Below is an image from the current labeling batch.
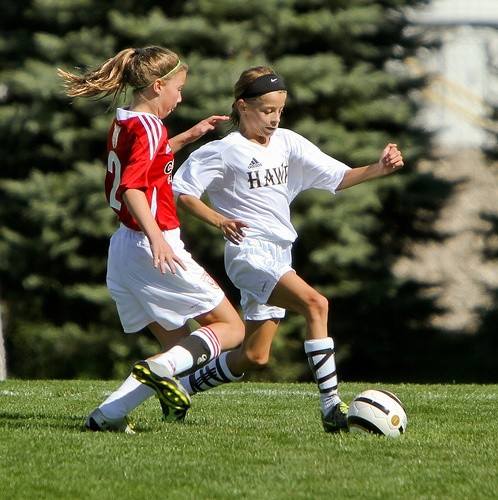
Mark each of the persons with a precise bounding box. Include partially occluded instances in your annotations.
[57,43,245,436]
[153,66,404,435]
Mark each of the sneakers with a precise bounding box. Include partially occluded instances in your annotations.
[85,407,143,436]
[157,396,186,423]
[130,360,192,412]
[319,401,348,433]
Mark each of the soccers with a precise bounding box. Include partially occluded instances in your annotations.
[347,389,407,436]
